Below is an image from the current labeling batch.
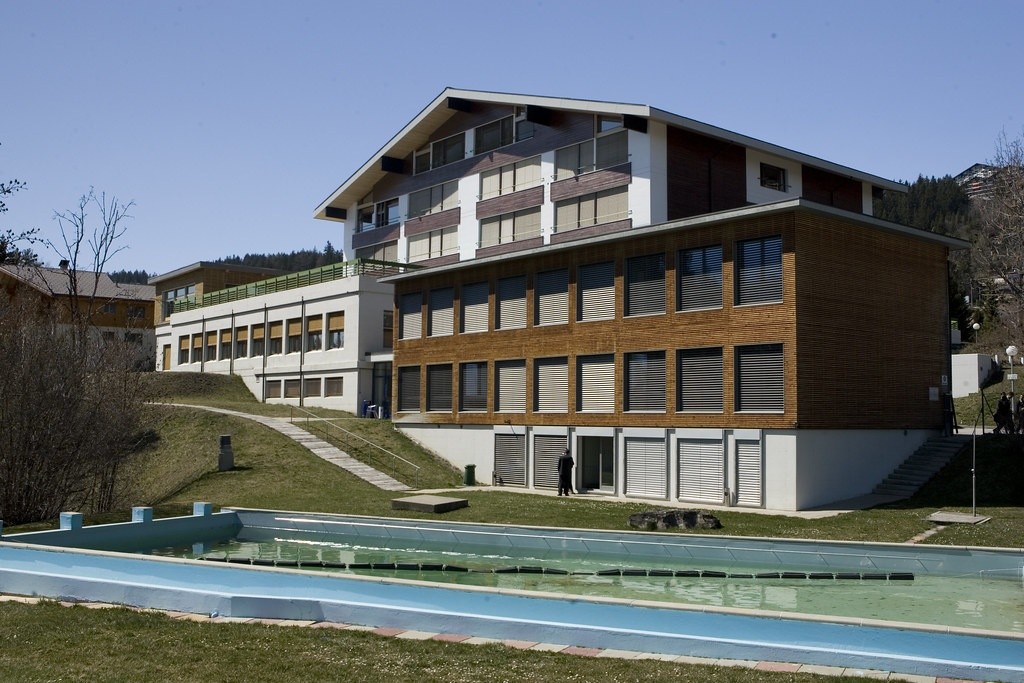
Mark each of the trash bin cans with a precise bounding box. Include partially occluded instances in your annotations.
[463,463,476,484]
[362,399,372,418]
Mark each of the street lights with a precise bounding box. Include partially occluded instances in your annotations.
[973,322,980,343]
[1005,345,1019,423]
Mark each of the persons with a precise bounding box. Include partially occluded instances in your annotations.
[994,392,1024,434]
[557,448,575,496]
